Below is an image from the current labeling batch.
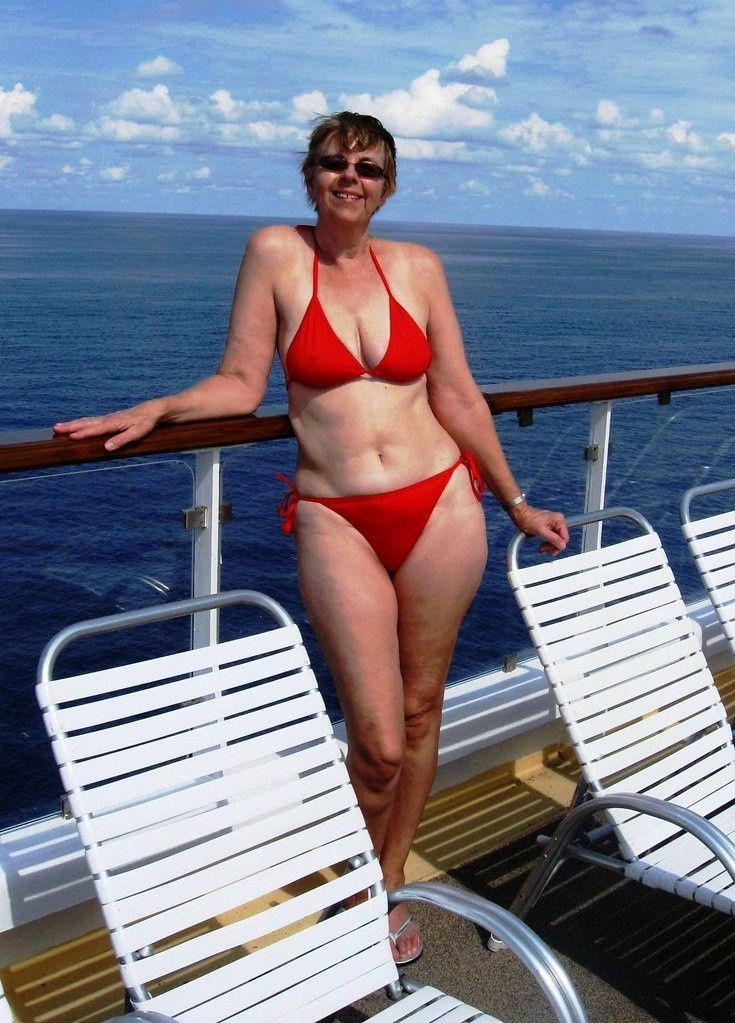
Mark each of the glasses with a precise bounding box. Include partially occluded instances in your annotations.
[310,155,388,178]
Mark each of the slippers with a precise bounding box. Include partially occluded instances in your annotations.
[388,913,423,966]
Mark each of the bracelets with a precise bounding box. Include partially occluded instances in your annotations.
[504,493,526,512]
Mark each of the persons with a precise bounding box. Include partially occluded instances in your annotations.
[51,114,572,966]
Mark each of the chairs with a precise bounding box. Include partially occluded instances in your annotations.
[680,478,735,655]
[485,507,735,953]
[33,593,584,1023]
